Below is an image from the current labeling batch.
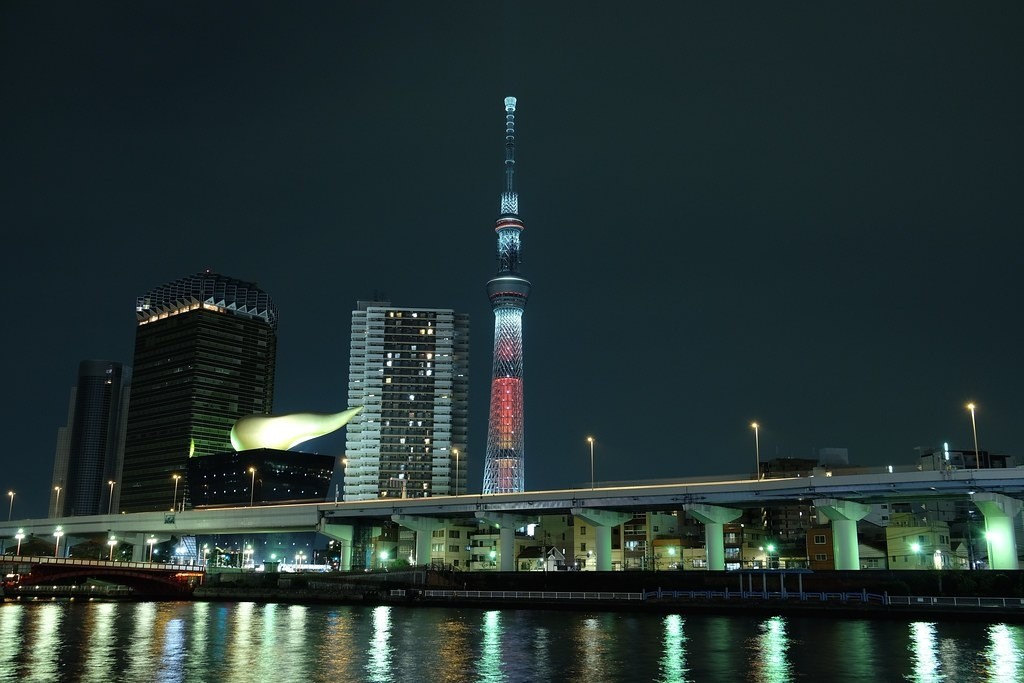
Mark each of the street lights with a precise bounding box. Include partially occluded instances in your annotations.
[295,550,306,567]
[751,422,760,480]
[14,528,25,555]
[768,544,775,568]
[107,535,117,560]
[488,551,497,569]
[452,447,458,496]
[52,525,65,557]
[983,530,998,570]
[108,480,117,515]
[147,534,158,563]
[244,544,254,567]
[8,491,16,520]
[588,437,593,490]
[342,458,348,502]
[172,474,182,511]
[909,542,920,571]
[55,486,62,518]
[248,467,255,506]
[667,546,676,563]
[379,550,388,569]
[202,544,209,565]
[966,403,981,468]
[176,546,188,565]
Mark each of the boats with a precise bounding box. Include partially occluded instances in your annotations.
[368,585,427,609]
